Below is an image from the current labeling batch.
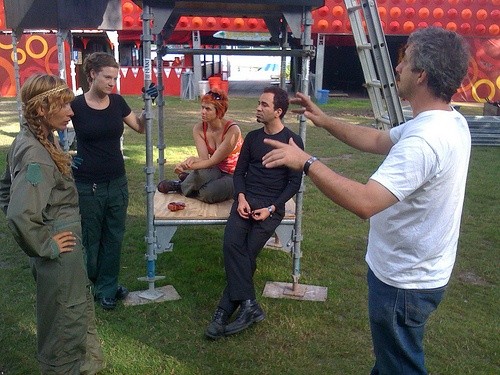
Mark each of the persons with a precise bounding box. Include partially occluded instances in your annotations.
[261,26,471,375]
[204,86,304,339]
[157,88,244,204]
[69,52,158,310]
[0,74,105,375]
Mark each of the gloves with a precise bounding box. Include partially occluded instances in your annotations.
[141,83,158,101]
[70,156,84,169]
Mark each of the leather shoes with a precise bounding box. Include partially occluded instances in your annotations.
[205,307,229,339]
[224,298,264,334]
[178,172,189,181]
[158,179,181,193]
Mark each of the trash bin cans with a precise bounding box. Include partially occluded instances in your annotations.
[317,90,330,104]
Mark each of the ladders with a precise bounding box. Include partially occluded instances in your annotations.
[341,0,409,130]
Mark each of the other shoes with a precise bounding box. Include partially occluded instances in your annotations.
[95,295,116,309]
[113,286,128,300]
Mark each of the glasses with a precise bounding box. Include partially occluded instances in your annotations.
[205,91,221,100]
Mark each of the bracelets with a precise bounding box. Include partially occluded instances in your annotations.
[268,206,273,217]
[304,157,319,176]
